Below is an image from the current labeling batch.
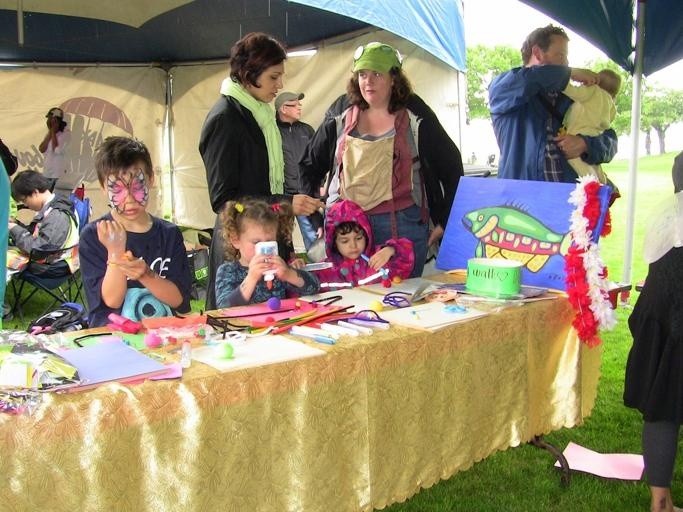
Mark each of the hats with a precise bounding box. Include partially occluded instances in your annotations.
[274,92,304,110]
[352,43,402,76]
[45,107,64,119]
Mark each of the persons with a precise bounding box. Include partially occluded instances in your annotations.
[198,31,327,217]
[78,136,191,328]
[288,199,416,297]
[214,201,321,309]
[2,170,80,321]
[296,41,465,279]
[274,92,327,253]
[38,107,72,178]
[622,150,683,512]
[487,23,618,183]
[562,69,620,184]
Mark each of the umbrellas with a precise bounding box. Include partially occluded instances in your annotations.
[59,96,133,157]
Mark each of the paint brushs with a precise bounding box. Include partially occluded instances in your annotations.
[360,253,388,275]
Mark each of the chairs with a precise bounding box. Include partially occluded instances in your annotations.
[0,193,90,324]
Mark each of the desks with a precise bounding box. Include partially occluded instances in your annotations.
[0,270,604,512]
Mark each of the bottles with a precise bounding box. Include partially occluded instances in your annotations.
[256,241,279,290]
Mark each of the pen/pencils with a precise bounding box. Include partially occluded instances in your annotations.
[250,305,390,344]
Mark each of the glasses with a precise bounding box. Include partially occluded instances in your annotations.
[286,103,302,108]
[21,193,30,204]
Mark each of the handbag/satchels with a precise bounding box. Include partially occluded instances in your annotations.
[0,140,18,176]
[28,302,89,335]
